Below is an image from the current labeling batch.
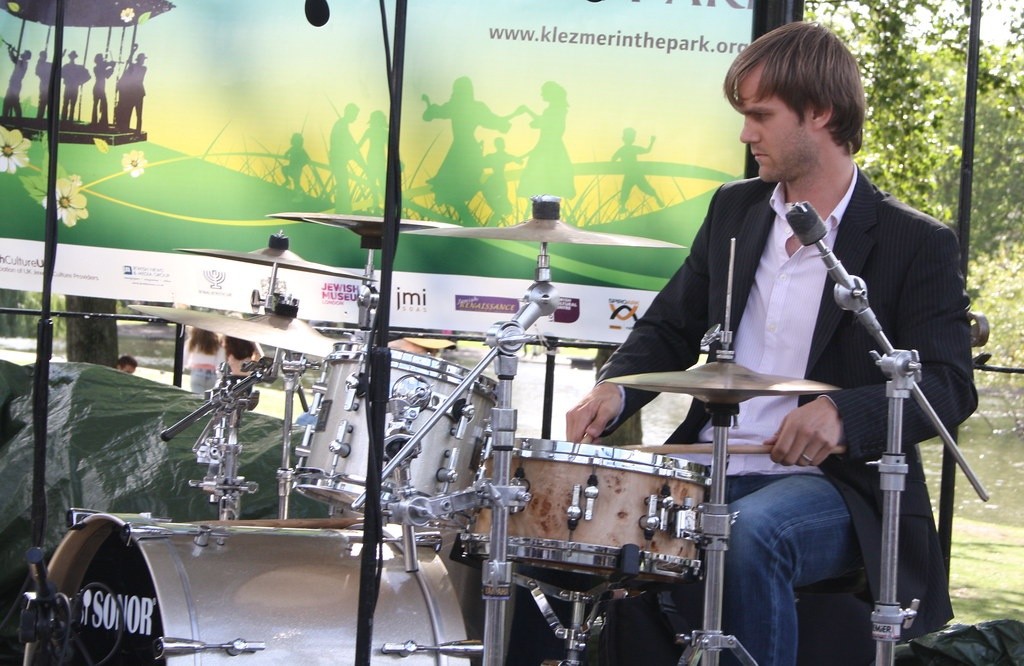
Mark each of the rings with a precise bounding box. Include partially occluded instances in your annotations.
[802,454,811,464]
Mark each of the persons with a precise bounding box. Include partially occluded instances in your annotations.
[565,21,980,666]
[115,356,138,374]
[183,312,265,397]
[389,336,456,357]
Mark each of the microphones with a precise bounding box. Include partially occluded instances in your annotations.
[786,201,853,288]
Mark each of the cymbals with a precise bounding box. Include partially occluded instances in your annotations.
[602,361,842,407]
[127,305,338,358]
[173,246,379,284]
[265,213,464,239]
[399,220,686,251]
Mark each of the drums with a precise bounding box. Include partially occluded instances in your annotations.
[23,513,473,666]
[291,341,499,529]
[461,438,712,581]
